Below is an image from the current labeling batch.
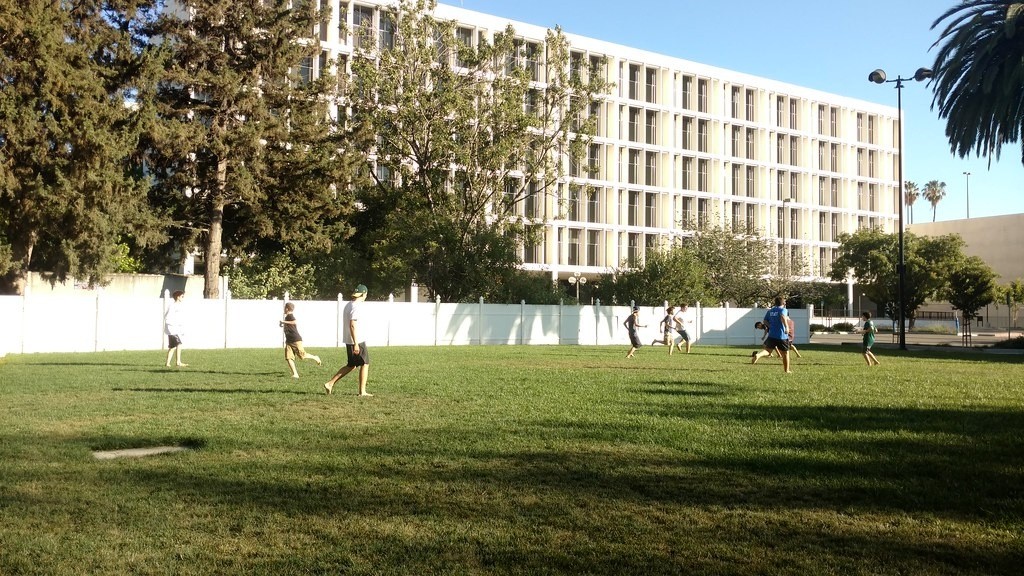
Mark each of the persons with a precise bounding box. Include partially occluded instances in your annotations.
[652,306,675,355]
[787,311,802,358]
[623,306,647,359]
[164,290,189,367]
[856,311,880,366]
[324,285,373,396]
[754,322,782,358]
[673,303,693,353]
[751,297,791,373]
[280,302,322,378]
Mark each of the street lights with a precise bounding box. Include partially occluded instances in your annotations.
[781,196,796,298]
[963,171,972,220]
[858,292,866,327]
[867,67,935,352]
[568,270,587,305]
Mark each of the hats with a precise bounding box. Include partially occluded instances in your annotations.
[351,284,367,298]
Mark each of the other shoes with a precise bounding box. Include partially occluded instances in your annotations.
[652,339,657,346]
[324,382,332,395]
[677,343,681,351]
[628,351,635,357]
[752,354,759,365]
[314,356,321,365]
[874,361,880,365]
